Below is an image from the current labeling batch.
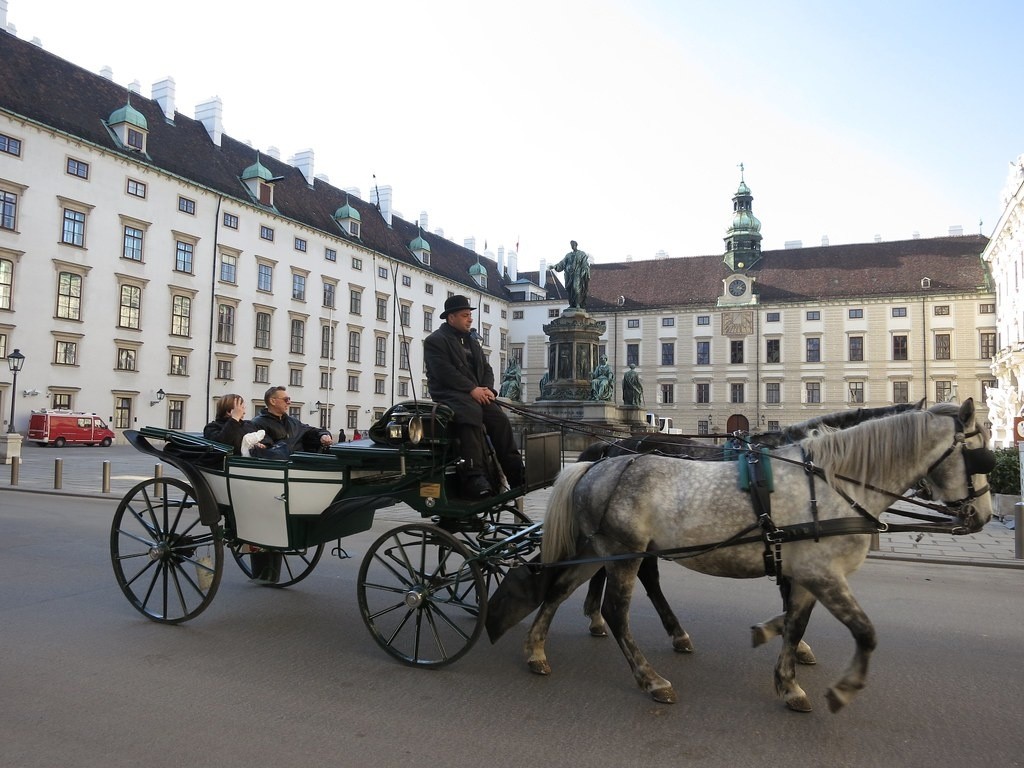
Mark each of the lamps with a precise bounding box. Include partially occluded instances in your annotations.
[708,414,712,425]
[761,415,765,424]
[150,388,165,407]
[310,400,322,415]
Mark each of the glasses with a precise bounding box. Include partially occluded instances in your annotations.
[271,397,291,402]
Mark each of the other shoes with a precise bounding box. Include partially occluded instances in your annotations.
[509,467,527,489]
[464,475,492,499]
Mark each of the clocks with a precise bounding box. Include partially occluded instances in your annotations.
[728,279,747,297]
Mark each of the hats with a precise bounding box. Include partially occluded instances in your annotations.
[440,295,477,319]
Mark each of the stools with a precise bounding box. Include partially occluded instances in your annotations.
[368,399,455,452]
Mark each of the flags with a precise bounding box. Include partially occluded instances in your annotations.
[516,242,519,252]
[485,241,488,249]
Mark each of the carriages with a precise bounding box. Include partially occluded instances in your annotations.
[108,377,996,712]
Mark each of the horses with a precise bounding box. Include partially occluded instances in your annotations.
[526,395,997,712]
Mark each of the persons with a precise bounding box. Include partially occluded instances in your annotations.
[338,429,346,443]
[548,240,590,308]
[498,356,522,400]
[353,430,361,440]
[252,387,332,453]
[424,295,527,497]
[588,354,614,401]
[622,363,643,405]
[203,394,272,457]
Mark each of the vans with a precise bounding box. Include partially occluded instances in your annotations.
[26,409,115,447]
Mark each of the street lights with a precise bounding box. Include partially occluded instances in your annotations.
[0,349,25,465]
